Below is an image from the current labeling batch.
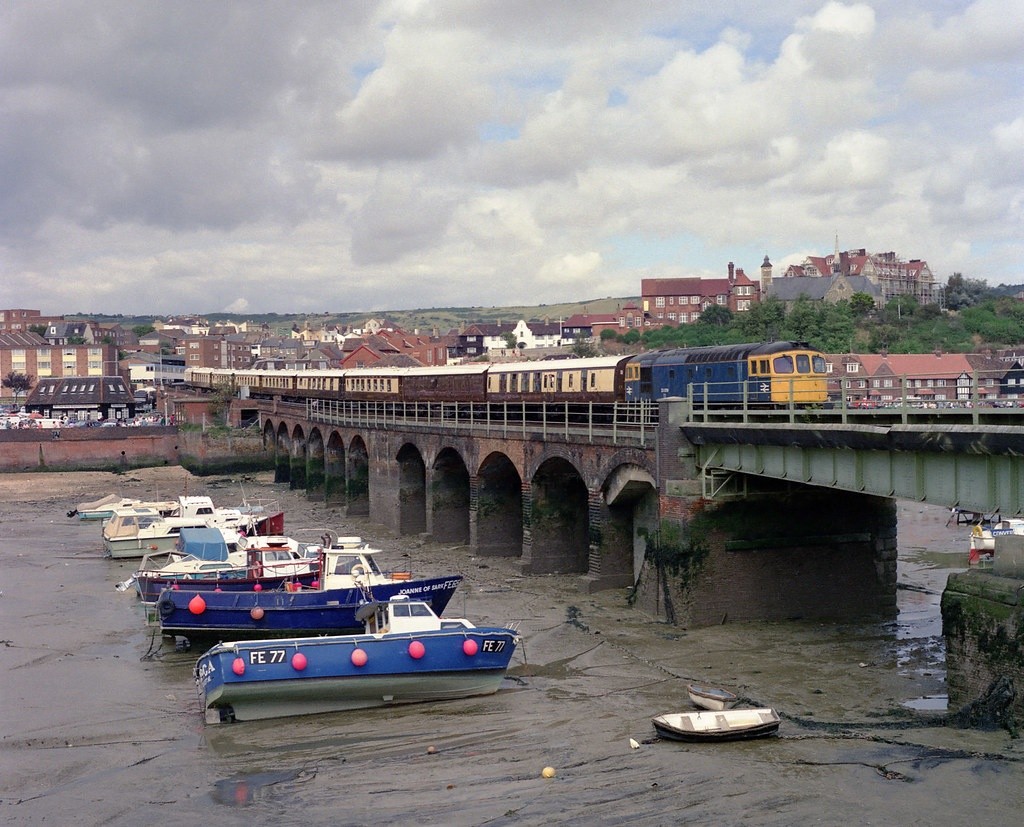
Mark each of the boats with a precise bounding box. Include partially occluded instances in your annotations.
[650,706,783,743]
[687,682,739,711]
[64,476,524,724]
[969,518,1024,562]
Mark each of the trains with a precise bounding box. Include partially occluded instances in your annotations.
[183,338,830,408]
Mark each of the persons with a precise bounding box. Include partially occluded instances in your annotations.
[4,413,176,430]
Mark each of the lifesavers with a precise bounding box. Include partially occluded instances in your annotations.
[389,569,413,582]
